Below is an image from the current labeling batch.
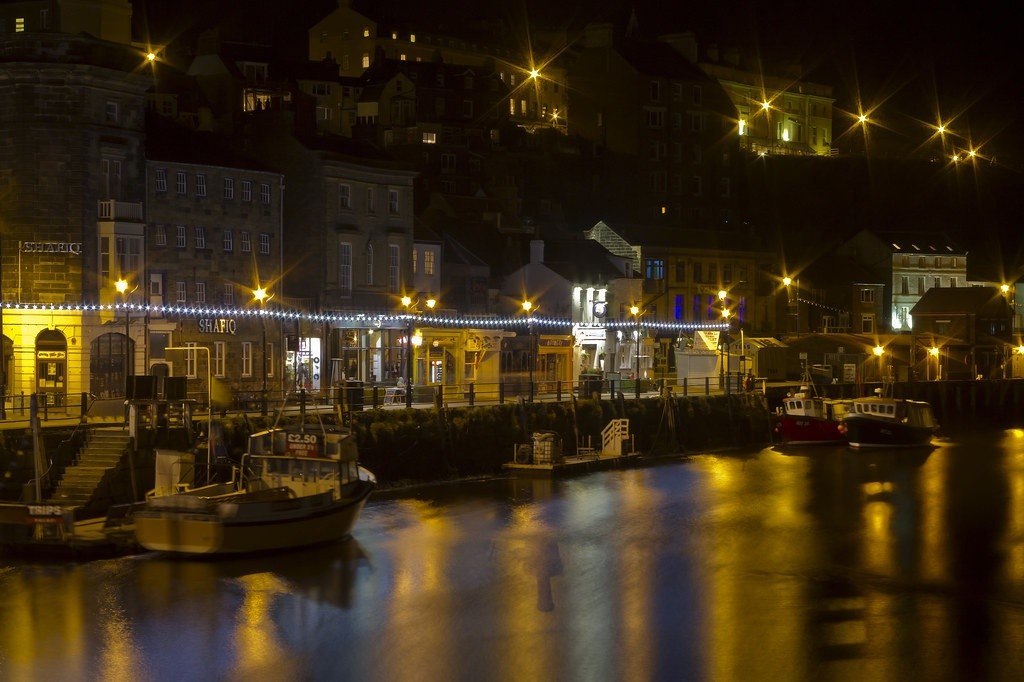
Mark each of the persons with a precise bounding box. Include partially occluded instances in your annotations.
[298,362,306,385]
[396,377,414,396]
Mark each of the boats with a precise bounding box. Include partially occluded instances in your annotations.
[834,366,943,453]
[130,337,378,555]
[772,351,842,448]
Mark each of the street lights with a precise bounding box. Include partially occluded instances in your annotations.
[401,293,414,407]
[630,300,645,399]
[716,288,730,393]
[113,277,131,425]
[519,300,537,402]
[253,284,270,417]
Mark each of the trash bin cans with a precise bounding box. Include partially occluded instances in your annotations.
[342,381,364,412]
[578,374,603,400]
